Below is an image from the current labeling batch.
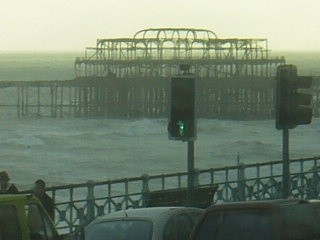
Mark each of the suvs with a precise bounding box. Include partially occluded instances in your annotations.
[0,194,60,240]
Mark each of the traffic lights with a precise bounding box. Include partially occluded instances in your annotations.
[168,78,196,139]
[276,65,310,130]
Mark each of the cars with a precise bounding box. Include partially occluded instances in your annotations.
[84,207,205,240]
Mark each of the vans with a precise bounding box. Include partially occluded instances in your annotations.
[189,200,320,240]
